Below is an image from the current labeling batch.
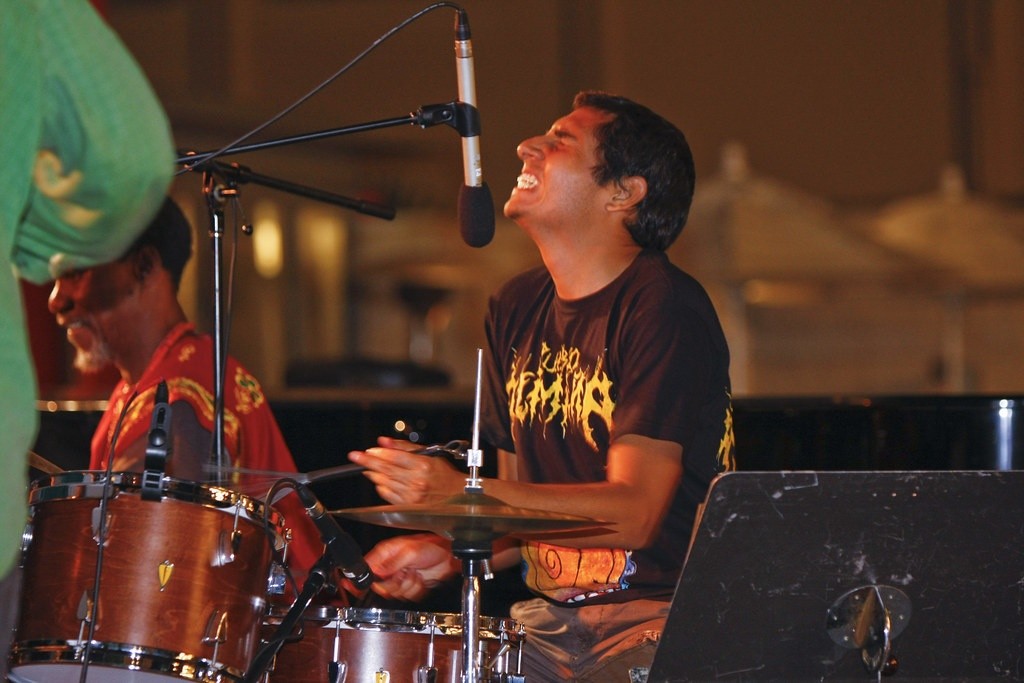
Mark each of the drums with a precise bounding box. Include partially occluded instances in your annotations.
[254,598,527,683]
[8,467,293,683]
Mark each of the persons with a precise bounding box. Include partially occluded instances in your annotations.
[46,192,322,606]
[348,91,738,683]
[0,0,176,683]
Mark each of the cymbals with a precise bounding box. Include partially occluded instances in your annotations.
[325,488,619,540]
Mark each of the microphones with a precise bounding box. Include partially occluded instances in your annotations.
[294,482,376,591]
[454,8,495,248]
[144,380,171,467]
